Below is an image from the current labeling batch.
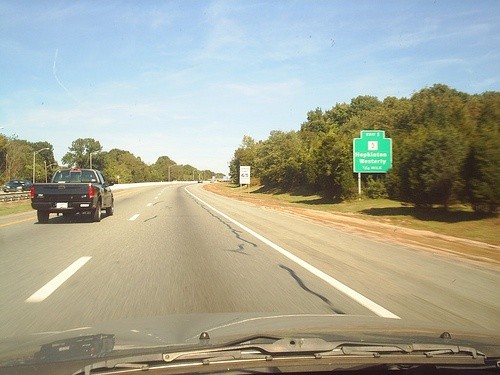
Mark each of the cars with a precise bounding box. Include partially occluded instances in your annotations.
[1,312,500,374]
[2,179,32,193]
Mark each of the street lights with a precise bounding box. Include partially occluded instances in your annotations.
[90,152,97,169]
[33,147,49,183]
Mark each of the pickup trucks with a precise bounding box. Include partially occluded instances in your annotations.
[31,168,114,222]
